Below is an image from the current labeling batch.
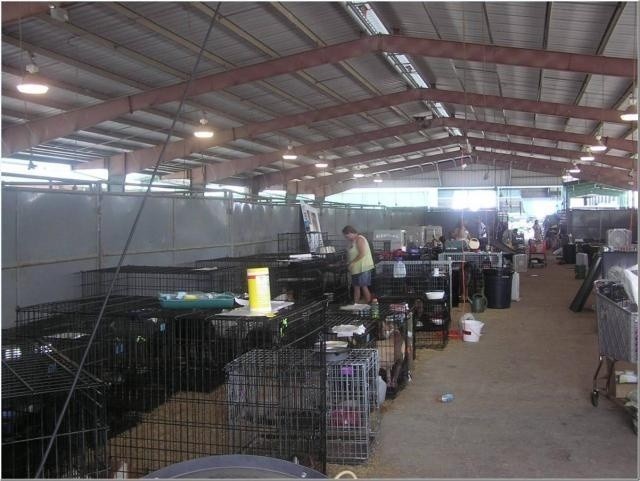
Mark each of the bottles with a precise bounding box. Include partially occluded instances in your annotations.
[371,298,380,319]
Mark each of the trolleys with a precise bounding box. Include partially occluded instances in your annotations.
[589,276,639,427]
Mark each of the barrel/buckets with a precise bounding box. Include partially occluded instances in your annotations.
[462,319,484,342]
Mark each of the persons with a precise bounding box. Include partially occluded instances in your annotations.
[449,221,519,243]
[531,219,542,241]
[341,225,375,304]
